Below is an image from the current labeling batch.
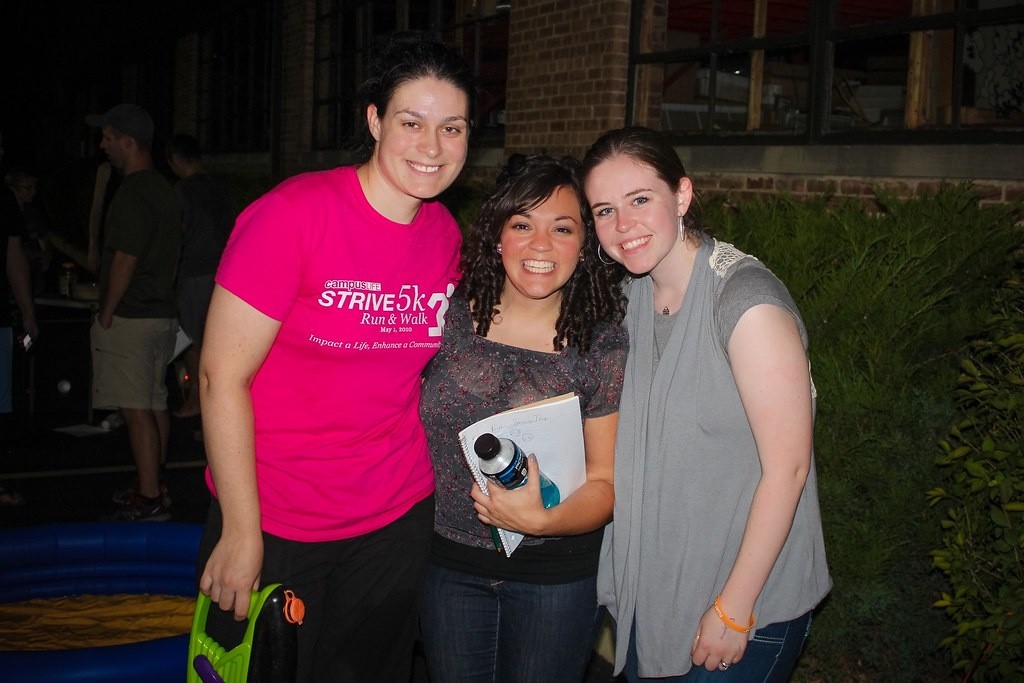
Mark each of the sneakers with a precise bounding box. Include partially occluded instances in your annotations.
[113,483,180,524]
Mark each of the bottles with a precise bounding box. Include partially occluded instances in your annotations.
[57,262,78,297]
[474,434,560,512]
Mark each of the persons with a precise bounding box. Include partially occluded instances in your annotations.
[581,126,833,683]
[0,104,224,524]
[198,40,480,683]
[417,153,631,683]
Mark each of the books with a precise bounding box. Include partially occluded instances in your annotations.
[458,391,587,558]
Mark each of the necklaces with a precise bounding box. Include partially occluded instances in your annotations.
[652,295,684,316]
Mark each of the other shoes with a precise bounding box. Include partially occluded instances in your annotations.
[1,486,28,509]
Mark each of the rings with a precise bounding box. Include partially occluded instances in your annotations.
[721,659,729,668]
[695,635,699,639]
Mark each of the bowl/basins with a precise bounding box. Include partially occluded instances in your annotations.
[69,284,100,300]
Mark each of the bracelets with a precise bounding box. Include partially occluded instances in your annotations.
[714,596,754,633]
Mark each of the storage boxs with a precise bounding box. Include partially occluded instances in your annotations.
[658,60,905,112]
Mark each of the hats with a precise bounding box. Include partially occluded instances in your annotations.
[85,103,154,143]
